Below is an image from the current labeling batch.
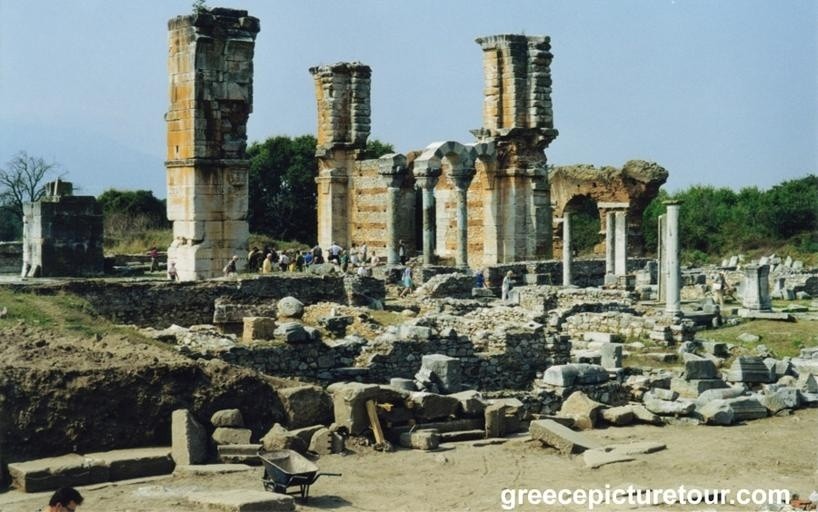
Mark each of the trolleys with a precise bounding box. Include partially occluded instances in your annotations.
[256,449,342,503]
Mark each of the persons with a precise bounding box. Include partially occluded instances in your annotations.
[475,269,488,290]
[398,238,407,265]
[399,262,416,298]
[501,270,514,301]
[41,485,84,511]
[149,245,161,272]
[169,260,179,281]
[223,242,378,274]
